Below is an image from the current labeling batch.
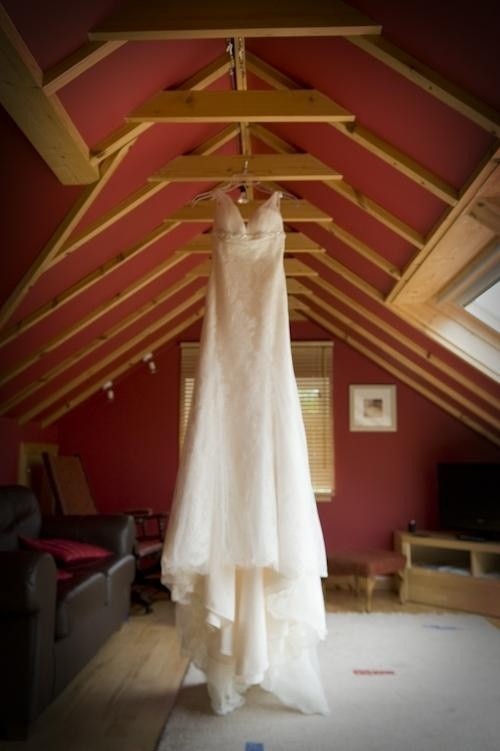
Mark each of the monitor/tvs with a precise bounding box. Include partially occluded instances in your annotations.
[432,456,500,540]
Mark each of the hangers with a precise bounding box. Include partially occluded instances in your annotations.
[187,159,301,212]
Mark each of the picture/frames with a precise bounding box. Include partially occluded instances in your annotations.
[348,382,398,434]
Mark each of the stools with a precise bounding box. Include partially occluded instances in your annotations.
[323,546,408,612]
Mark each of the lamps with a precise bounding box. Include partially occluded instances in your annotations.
[141,351,158,374]
[102,381,115,400]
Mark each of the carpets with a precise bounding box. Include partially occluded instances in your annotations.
[153,607,499,751]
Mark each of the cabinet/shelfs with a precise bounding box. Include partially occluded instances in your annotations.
[391,526,500,621]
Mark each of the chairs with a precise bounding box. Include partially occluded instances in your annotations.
[0,484,140,751]
[40,449,173,619]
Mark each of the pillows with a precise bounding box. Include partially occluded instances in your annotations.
[14,527,114,572]
[52,569,84,585]
